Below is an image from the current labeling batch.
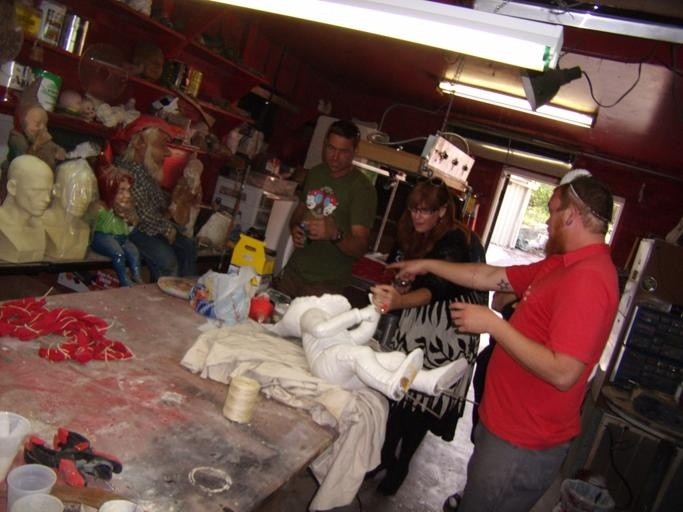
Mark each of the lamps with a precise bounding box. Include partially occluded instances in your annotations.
[437,79,593,127]
[209,1,563,72]
[522,56,648,110]
[447,127,575,170]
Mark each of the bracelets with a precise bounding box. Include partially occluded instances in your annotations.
[330,229,345,246]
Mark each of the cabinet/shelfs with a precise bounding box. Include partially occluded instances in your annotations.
[213,176,298,276]
[0,0,271,165]
[350,133,476,294]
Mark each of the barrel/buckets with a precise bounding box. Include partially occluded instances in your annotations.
[197,210,232,251]
[37,67,61,114]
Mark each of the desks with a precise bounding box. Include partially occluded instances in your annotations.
[1,285,338,512]
[0,250,221,276]
[577,384,683,512]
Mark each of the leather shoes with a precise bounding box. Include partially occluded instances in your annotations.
[365,454,408,496]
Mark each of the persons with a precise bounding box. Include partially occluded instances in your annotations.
[288,288,467,403]
[361,174,486,497]
[279,119,378,301]
[383,173,620,511]
[0,102,204,285]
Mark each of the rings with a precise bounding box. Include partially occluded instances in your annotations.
[380,303,388,312]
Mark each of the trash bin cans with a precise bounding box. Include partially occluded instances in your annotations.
[560,479,614,512]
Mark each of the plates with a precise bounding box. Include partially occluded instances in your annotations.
[157,276,193,299]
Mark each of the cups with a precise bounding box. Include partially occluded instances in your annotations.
[8,495,65,512]
[98,498,138,511]
[5,463,56,509]
[0,410,30,482]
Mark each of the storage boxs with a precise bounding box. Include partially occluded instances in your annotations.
[228,234,277,289]
[252,171,298,196]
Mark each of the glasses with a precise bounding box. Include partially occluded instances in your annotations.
[416,174,444,188]
[408,204,436,214]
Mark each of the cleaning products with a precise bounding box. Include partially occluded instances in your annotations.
[143,45,203,97]
